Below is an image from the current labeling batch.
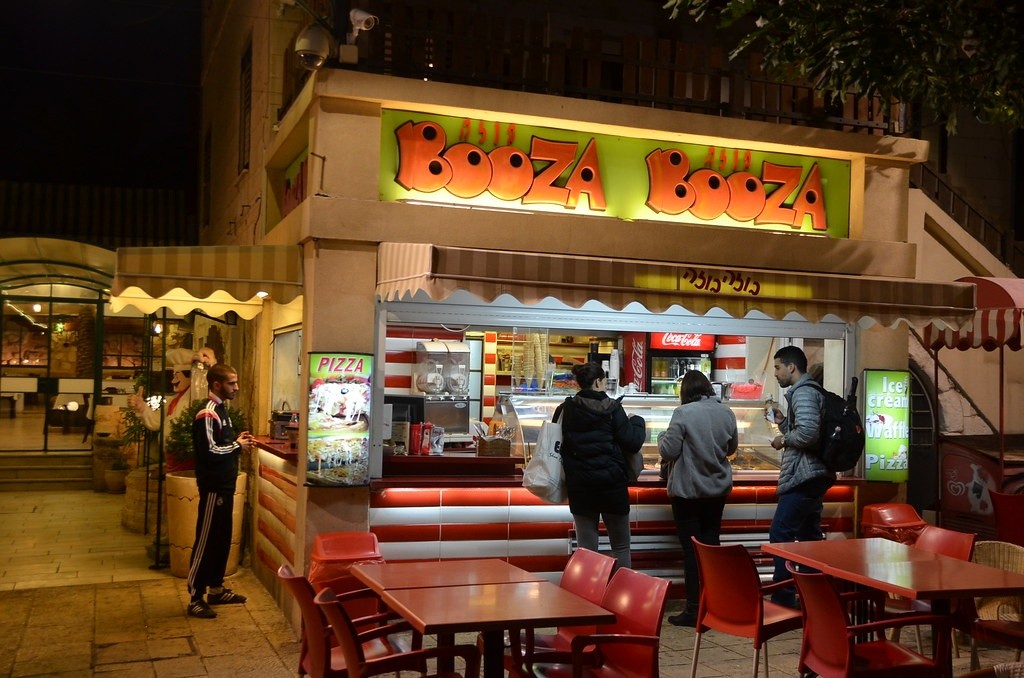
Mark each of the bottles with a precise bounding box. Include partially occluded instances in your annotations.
[652,354,712,400]
[288,410,298,426]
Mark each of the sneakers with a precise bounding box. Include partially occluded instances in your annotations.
[207,589,247,607]
[187,599,217,618]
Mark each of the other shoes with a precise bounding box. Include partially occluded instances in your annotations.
[668,612,697,628]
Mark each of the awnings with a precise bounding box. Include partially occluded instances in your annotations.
[376,240,978,333]
[923,274,1024,495]
[109,244,304,322]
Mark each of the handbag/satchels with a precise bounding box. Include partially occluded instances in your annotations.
[659,457,672,478]
[522,408,566,504]
[618,449,644,485]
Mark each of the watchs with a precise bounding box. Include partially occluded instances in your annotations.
[780,437,786,446]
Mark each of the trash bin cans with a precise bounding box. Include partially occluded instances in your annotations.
[860,503,927,546]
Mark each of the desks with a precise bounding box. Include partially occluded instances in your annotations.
[379,580,616,678]
[58,379,144,435]
[822,559,1021,678]
[350,557,548,675]
[760,538,933,677]
[1,377,38,417]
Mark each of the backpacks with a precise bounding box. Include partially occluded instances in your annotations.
[790,383,864,472]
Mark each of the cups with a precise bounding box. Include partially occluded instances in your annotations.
[602,348,619,380]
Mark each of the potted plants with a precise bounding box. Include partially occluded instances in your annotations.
[105,455,131,491]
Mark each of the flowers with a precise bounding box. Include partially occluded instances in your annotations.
[169,404,253,478]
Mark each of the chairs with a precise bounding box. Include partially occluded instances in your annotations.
[690,525,1024,678]
[278,547,673,678]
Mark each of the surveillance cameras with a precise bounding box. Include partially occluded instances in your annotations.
[350,9,380,29]
[295,21,331,71]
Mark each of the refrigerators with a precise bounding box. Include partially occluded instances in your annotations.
[621,331,719,467]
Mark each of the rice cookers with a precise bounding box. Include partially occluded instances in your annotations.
[268,401,299,440]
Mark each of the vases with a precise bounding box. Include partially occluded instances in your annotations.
[166,469,249,578]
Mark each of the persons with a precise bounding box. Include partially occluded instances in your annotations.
[657,370,740,626]
[127,348,218,545]
[762,345,840,608]
[552,362,647,573]
[187,363,261,617]
